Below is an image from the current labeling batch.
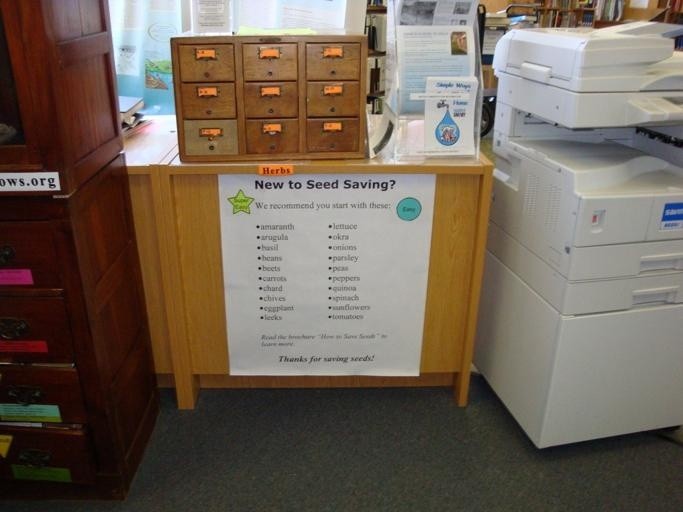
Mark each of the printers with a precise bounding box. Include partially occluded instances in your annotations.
[473,19,683,451]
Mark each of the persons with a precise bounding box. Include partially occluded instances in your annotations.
[451,33,467,54]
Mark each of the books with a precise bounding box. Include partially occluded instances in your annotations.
[546,1,593,28]
[484,4,538,28]
